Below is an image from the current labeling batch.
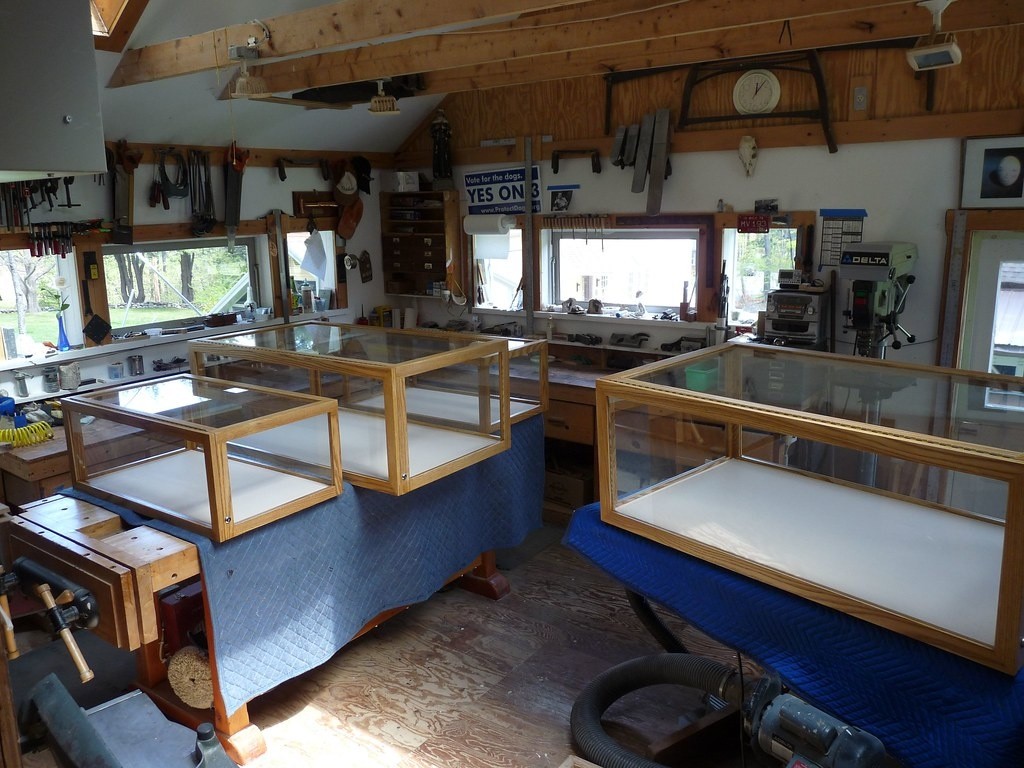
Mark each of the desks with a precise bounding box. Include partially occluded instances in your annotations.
[0,332,510,518]
[342,333,787,507]
[562,500,1024,768]
[0,375,549,766]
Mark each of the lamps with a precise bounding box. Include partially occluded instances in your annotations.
[368,77,400,116]
[229,44,273,98]
[907,0,961,72]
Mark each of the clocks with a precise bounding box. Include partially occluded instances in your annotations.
[733,69,780,116]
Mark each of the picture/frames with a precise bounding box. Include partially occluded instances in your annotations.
[958,133,1024,210]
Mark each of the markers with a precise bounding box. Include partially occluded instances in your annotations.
[666,312,679,321]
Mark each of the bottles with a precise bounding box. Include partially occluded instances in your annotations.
[289,276,301,309]
[244,286,256,321]
[301,278,313,312]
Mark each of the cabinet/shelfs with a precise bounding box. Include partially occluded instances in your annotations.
[188,320,512,496]
[337,324,551,433]
[592,340,1024,675]
[378,191,463,299]
[59,372,344,544]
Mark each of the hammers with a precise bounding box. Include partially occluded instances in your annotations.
[299,198,339,213]
[257,208,294,324]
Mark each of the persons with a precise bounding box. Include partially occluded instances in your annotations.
[553,191,568,212]
